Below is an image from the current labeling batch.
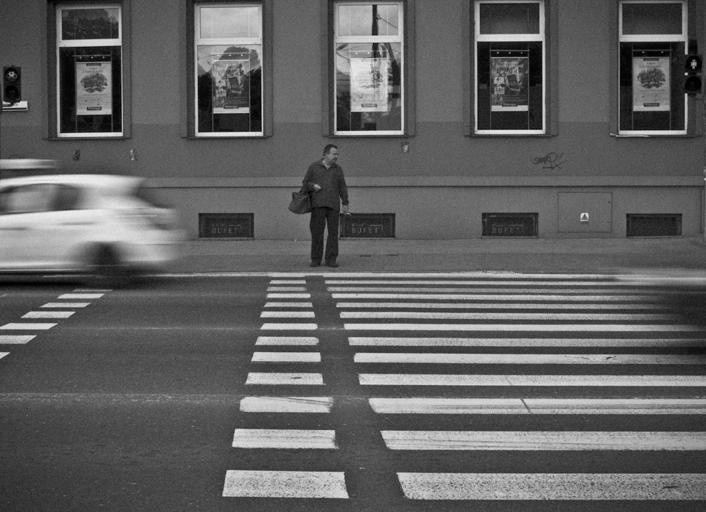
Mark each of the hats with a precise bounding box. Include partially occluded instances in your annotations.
[1,175,188,286]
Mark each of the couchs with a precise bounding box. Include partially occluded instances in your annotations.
[683,53,702,95]
[4,64,23,103]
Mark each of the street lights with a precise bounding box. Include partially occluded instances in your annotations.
[289,191,312,213]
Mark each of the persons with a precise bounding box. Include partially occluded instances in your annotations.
[302,145,349,268]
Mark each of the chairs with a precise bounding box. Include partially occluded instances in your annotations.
[310,262,320,266]
[325,263,339,267]
